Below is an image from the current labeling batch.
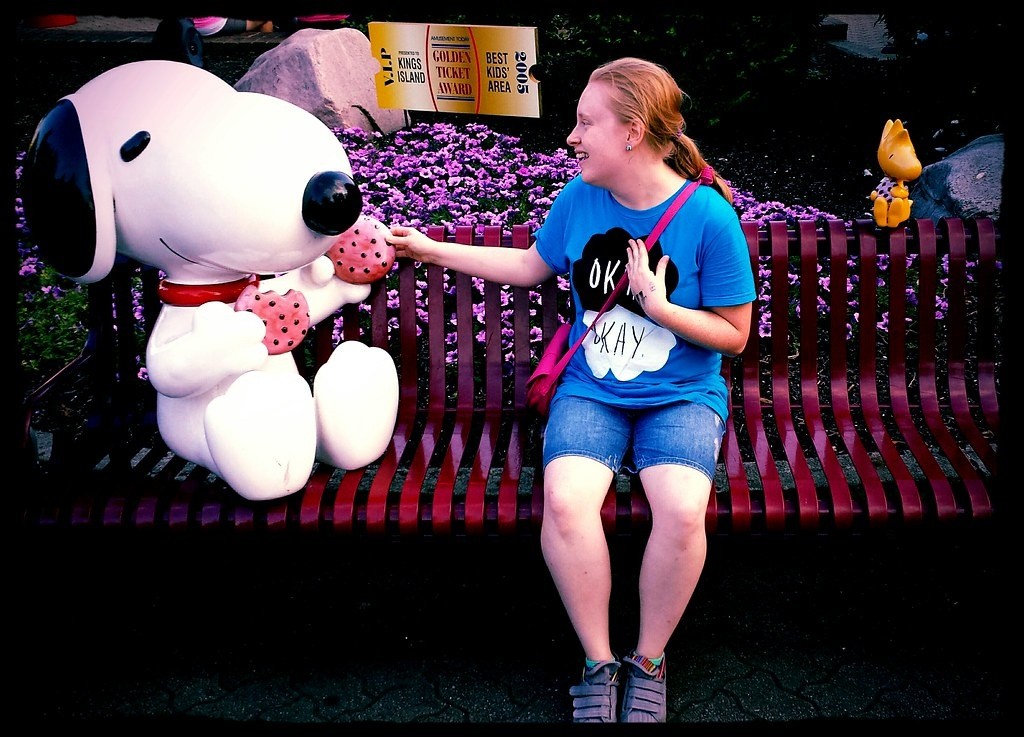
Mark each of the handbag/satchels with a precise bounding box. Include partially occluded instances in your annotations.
[525,323,571,418]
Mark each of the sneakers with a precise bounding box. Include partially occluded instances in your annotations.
[622,649,667,722]
[569,650,622,723]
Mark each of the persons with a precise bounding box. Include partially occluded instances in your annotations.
[193,16,273,36]
[385,58,757,723]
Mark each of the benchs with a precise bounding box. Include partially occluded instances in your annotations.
[17,217,1003,556]
